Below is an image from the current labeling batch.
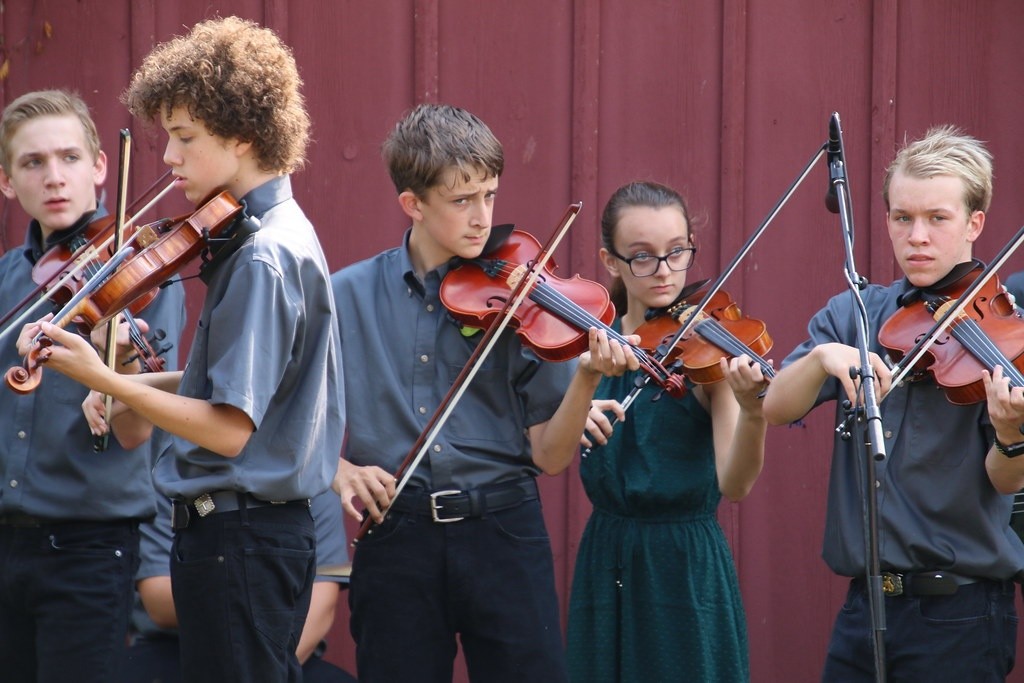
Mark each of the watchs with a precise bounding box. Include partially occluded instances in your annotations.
[993,432,1024,459]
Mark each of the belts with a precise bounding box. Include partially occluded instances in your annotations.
[377,476,540,523]
[1,513,60,530]
[169,490,312,529]
[866,567,982,598]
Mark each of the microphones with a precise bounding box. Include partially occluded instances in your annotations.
[826,112,849,213]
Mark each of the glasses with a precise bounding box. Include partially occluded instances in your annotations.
[608,239,697,277]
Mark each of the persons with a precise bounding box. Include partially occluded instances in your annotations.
[332,98,638,683]
[141,423,359,682]
[764,124,1023,683]
[14,20,333,683]
[1,91,187,683]
[566,184,770,682]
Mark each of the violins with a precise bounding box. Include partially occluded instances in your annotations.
[3,188,245,397]
[628,288,809,432]
[29,211,176,374]
[878,254,1022,408]
[438,225,687,406]
